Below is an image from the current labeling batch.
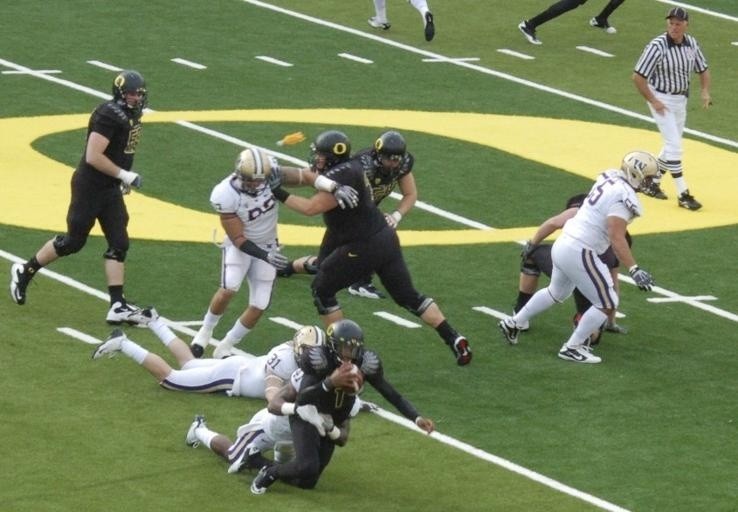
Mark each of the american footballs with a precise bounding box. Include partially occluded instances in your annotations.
[345,365,363,396]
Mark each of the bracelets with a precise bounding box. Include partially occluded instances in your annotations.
[273,187,290,202]
[391,211,402,222]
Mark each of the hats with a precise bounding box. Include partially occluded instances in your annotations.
[665,7,688,20]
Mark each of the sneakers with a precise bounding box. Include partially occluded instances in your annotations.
[678,189,701,211]
[347,281,387,299]
[643,183,666,199]
[451,309,614,366]
[212,343,234,358]
[276,264,293,279]
[365,11,616,45]
[10,262,158,360]
[189,326,214,358]
[185,415,276,495]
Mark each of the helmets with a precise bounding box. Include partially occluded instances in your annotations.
[309,131,350,172]
[376,131,406,177]
[293,326,326,354]
[620,152,658,193]
[236,148,272,196]
[113,71,147,119]
[325,318,365,369]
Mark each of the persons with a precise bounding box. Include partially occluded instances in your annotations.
[514,194,632,334]
[276,130,417,299]
[272,131,472,365]
[518,0,625,45]
[185,319,434,494]
[497,152,661,363]
[367,0,435,41]
[633,7,712,210]
[189,148,360,359]
[9,70,144,325]
[93,308,327,402]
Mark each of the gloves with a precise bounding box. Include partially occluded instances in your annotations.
[267,245,289,271]
[627,264,655,291]
[384,211,402,229]
[118,167,143,194]
[520,240,536,262]
[295,405,318,425]
[602,323,626,334]
[332,183,360,210]
